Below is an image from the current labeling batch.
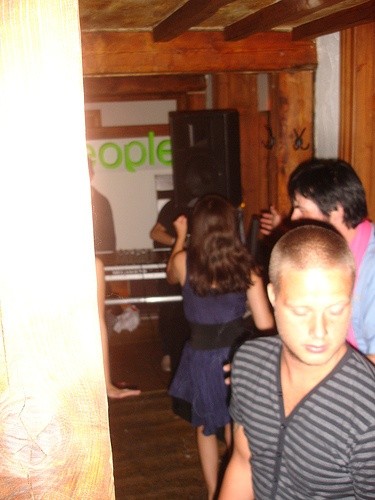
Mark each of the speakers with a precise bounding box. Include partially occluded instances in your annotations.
[169,108,241,212]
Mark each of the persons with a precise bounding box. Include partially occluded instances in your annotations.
[166,195,275,500]
[255,203,281,236]
[217,224,375,499]
[221,217,348,387]
[149,193,203,372]
[94,257,141,399]
[88,155,117,254]
[287,157,375,365]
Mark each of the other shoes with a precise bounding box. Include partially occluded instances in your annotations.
[161,354,172,372]
